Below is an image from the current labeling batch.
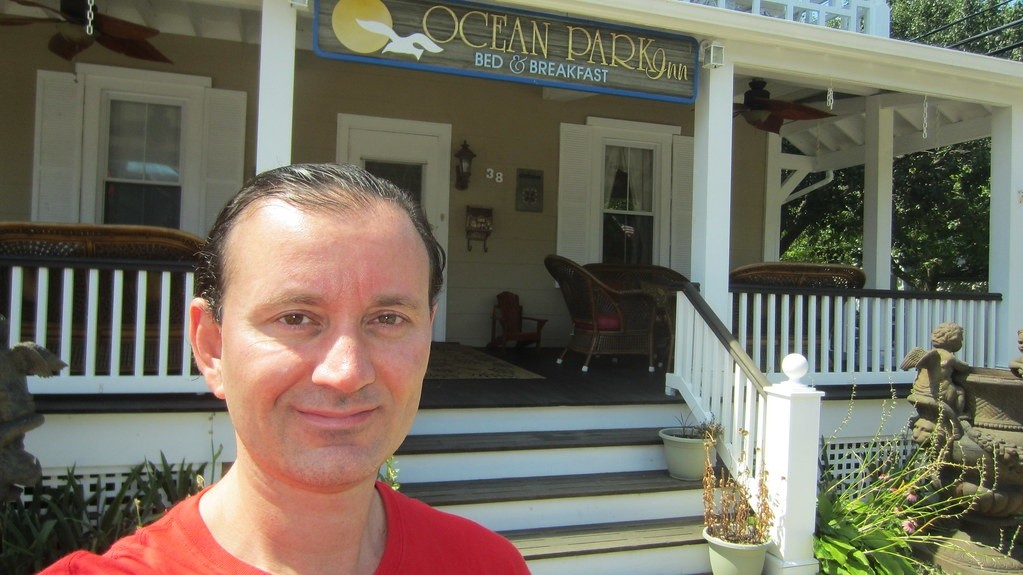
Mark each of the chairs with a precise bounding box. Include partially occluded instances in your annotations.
[0,217,207,364]
[490,291,547,358]
[544,254,661,373]
[584,262,699,349]
[638,278,681,373]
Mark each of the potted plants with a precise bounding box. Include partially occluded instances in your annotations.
[659,404,720,480]
[702,461,771,575]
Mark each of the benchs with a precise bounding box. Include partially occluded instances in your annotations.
[727,260,867,361]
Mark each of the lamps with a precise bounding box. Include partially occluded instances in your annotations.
[455,140,477,191]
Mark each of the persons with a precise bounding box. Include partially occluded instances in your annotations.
[37,161,534,575]
[0,314,68,454]
[899,323,972,429]
[1008,329,1023,379]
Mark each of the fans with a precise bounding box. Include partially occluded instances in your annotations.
[733,75,839,133]
[1,0,176,66]
